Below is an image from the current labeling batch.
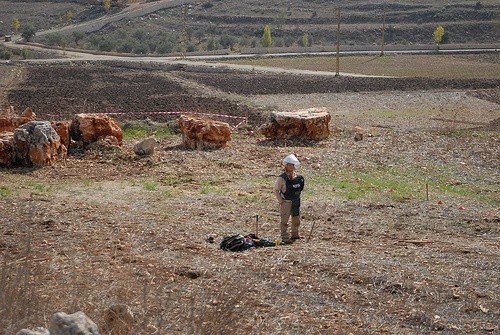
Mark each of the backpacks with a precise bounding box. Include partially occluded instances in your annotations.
[220,234,246,252]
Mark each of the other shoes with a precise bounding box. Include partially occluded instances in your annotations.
[282,239,292,244]
[291,235,305,240]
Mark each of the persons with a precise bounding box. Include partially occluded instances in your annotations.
[274,155,303,243]
